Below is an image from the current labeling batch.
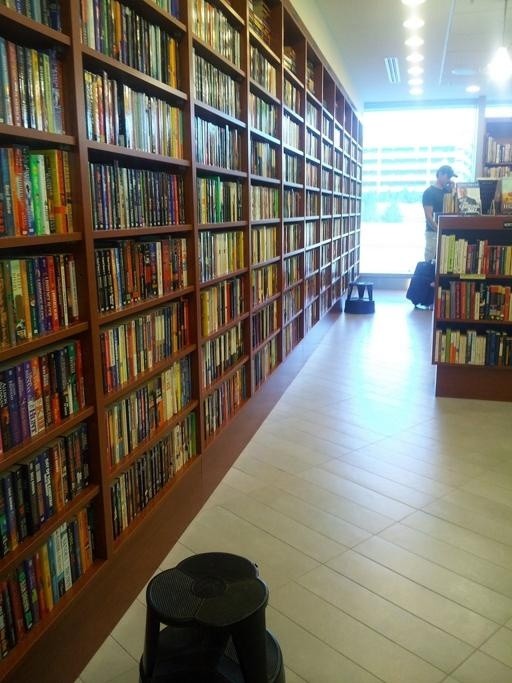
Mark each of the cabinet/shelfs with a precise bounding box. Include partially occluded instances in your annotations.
[472,95,512,214]
[75,1,205,621]
[431,215,512,402]
[0,1,113,683]
[248,1,362,442]
[190,2,250,526]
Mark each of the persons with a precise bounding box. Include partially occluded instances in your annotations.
[457,187,477,215]
[423,165,458,263]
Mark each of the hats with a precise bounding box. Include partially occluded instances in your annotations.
[436,165,458,178]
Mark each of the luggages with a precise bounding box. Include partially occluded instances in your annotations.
[406,251,436,311]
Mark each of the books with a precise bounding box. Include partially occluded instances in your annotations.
[0,145,74,236]
[434,328,512,365]
[489,178,512,215]
[89,163,186,231]
[201,277,247,336]
[82,1,178,88]
[437,280,512,322]
[0,423,89,557]
[3,1,68,34]
[202,322,247,388]
[0,40,66,138]
[193,47,241,118]
[111,411,198,541]
[192,0,241,70]
[84,68,184,160]
[195,116,243,172]
[0,510,94,660]
[197,175,243,223]
[101,298,192,394]
[199,231,246,282]
[439,233,510,276]
[204,366,249,442]
[0,338,86,453]
[95,237,187,314]
[110,355,195,465]
[442,193,457,214]
[483,136,512,178]
[155,0,179,18]
[0,253,79,346]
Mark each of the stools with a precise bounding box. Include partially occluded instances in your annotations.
[345,282,375,313]
[139,553,284,682]
